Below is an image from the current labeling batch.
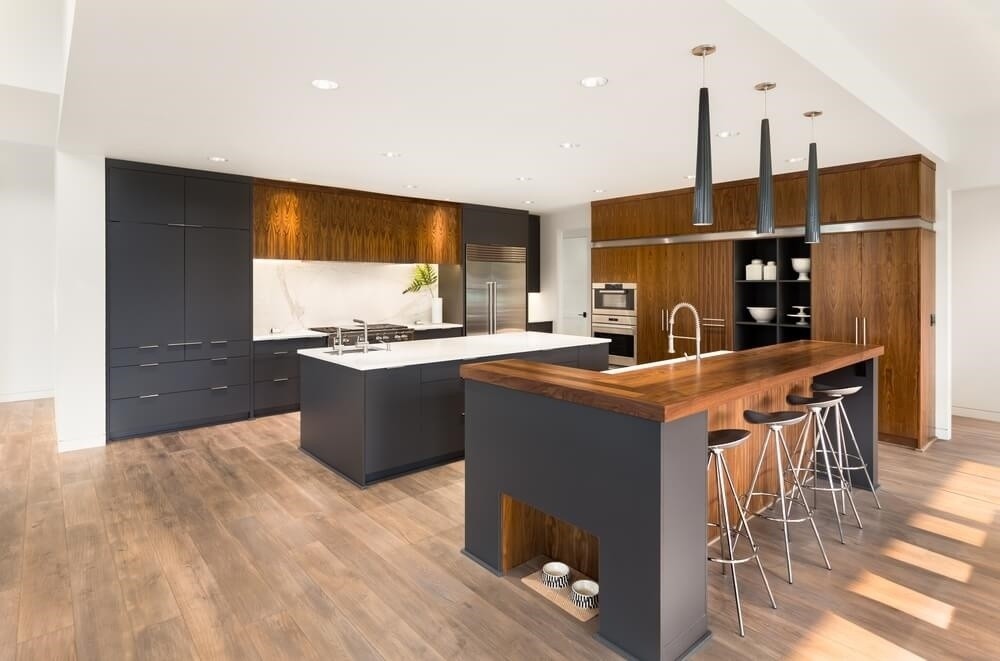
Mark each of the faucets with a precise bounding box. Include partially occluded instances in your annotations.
[667,302,701,361]
[333,328,344,356]
[352,318,369,353]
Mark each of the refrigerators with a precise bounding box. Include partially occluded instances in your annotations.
[466,243,527,338]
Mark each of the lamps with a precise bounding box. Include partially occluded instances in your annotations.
[754,81,777,234]
[806,112,820,244]
[692,45,716,226]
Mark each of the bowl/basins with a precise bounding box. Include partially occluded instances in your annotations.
[746,307,776,322]
[541,561,571,589]
[569,579,599,609]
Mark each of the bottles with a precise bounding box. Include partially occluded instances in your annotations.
[764,262,776,280]
[745,260,764,280]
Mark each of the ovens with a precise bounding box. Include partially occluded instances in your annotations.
[591,281,637,367]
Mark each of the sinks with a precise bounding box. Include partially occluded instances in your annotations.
[600,349,736,375]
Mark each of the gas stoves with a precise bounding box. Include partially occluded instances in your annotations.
[309,322,409,334]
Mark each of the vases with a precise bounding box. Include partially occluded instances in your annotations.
[432,298,442,324]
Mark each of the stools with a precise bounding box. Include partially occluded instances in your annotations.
[705,381,881,637]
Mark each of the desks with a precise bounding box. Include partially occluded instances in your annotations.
[459,339,885,661]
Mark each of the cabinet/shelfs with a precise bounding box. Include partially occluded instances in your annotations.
[636,240,734,365]
[589,153,938,241]
[811,228,936,452]
[104,156,253,442]
[733,235,810,350]
[253,328,611,491]
